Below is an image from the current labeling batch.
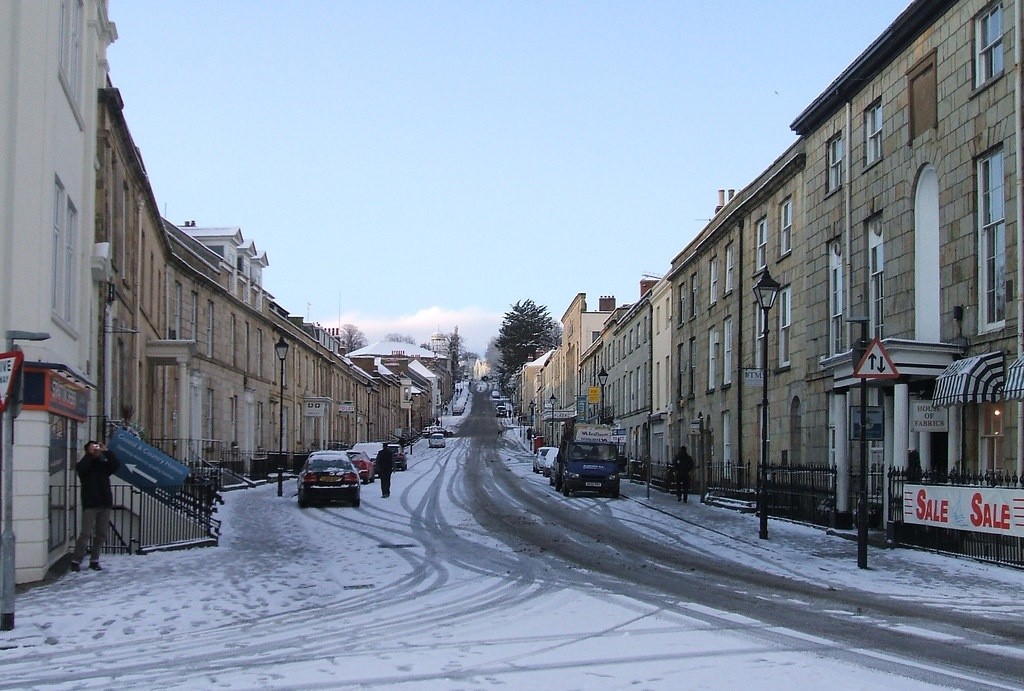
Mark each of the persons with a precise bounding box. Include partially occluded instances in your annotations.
[399,434,404,451]
[375,443,396,498]
[70,441,120,571]
[499,408,532,440]
[672,446,693,503]
[432,418,440,426]
[456,388,463,395]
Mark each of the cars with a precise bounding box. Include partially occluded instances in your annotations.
[496,406,508,418]
[532,446,552,474]
[543,448,559,476]
[352,442,383,475]
[492,391,500,399]
[422,425,453,439]
[549,457,559,486]
[296,449,374,508]
[386,444,408,472]
[428,433,446,448]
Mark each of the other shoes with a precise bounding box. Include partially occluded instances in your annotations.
[678,499,681,502]
[684,500,687,503]
[380,494,390,498]
[89,561,102,572]
[71,562,80,572]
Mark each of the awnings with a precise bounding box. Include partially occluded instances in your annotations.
[931,351,1005,409]
[1002,357,1024,402]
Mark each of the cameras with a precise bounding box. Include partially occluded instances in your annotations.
[93,444,99,449]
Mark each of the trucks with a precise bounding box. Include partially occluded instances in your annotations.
[553,438,629,498]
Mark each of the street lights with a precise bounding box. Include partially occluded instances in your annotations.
[274,334,290,496]
[597,365,609,424]
[427,399,432,435]
[529,401,535,450]
[409,397,414,455]
[365,380,373,444]
[549,393,558,448]
[752,264,783,540]
